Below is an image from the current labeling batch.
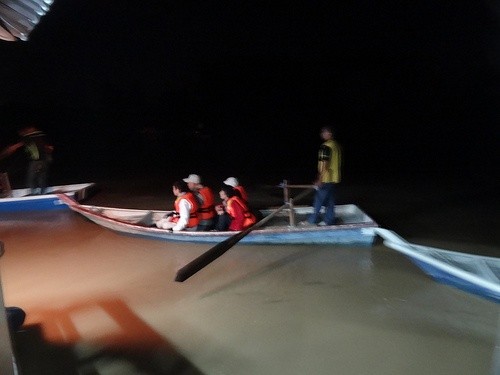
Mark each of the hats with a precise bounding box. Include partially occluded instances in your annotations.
[183,174,201,183]
[223,177,239,187]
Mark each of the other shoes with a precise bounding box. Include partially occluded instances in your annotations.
[300,220,311,225]
[318,221,327,226]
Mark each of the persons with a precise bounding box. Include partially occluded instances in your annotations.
[4,128,53,196]
[183,174,215,230]
[149,180,199,231]
[299,127,341,225]
[208,177,257,231]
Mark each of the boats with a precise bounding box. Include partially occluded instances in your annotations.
[0,182,99,214]
[370,227,500,304]
[54,189,379,245]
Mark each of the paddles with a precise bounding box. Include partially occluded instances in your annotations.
[174,187,315,284]
